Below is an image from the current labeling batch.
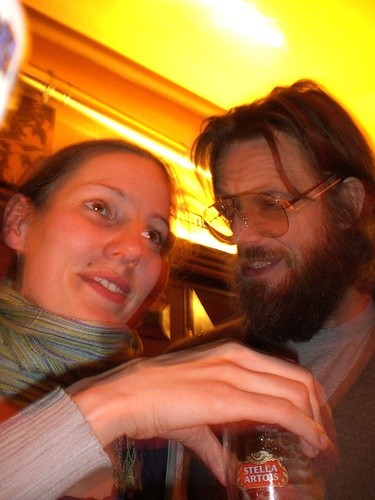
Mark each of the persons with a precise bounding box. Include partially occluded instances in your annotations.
[147,79,375,500]
[0,137,334,500]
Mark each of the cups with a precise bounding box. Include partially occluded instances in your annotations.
[222,420,326,500]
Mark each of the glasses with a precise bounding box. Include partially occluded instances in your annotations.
[201,174,341,244]
